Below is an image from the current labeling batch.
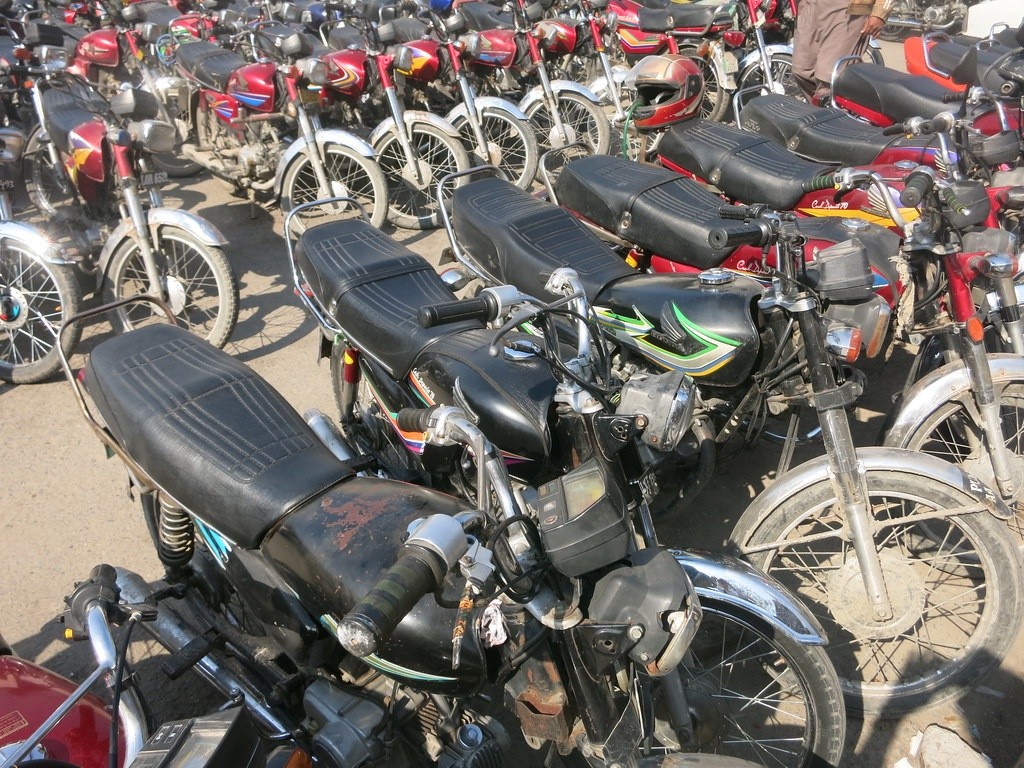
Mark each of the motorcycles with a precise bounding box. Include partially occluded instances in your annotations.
[1,0,1024,768]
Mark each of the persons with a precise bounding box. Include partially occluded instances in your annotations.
[792,0,894,103]
